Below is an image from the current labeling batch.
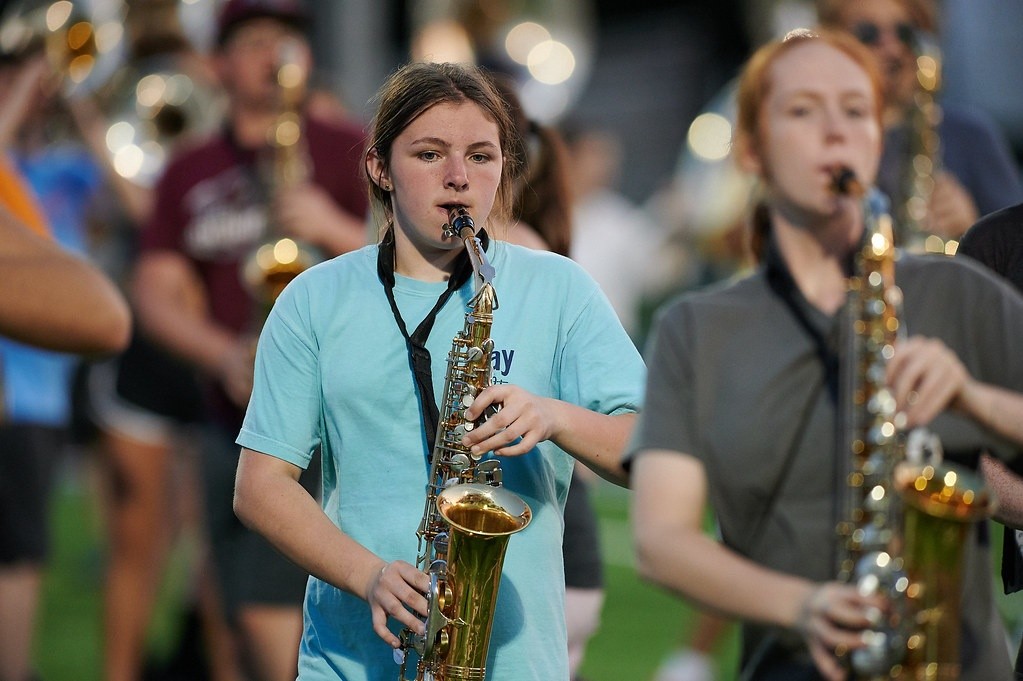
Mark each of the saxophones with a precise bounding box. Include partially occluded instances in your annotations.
[831,165,1001,681]
[395,202,534,681]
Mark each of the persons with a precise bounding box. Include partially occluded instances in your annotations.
[232,63,649,681]
[0,0,1023,681]
[619,28,1023,681]
